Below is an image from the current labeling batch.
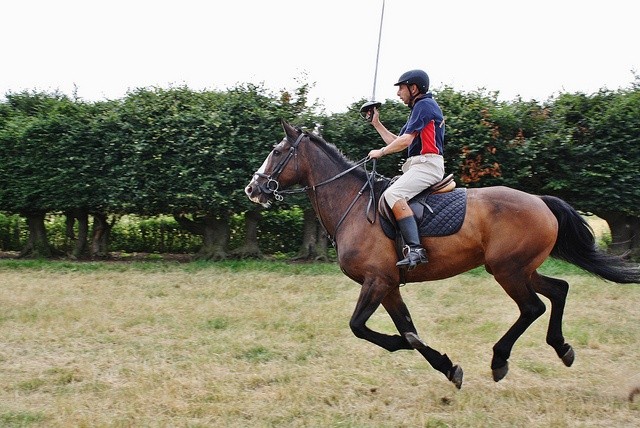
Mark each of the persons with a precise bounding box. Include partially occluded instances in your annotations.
[364,69,446,267]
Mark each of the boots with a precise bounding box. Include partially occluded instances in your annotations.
[391,198,429,268]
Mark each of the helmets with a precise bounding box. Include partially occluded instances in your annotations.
[394,70,429,94]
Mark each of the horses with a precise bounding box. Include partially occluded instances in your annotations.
[243,118,640,390]
